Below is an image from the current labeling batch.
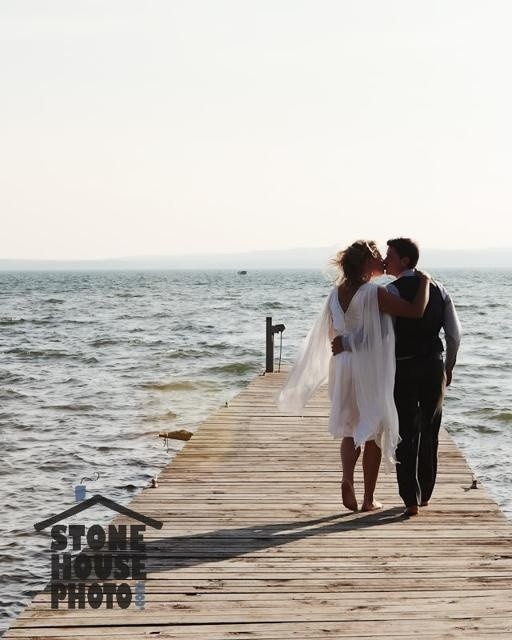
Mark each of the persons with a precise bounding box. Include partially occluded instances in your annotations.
[330,237,461,517]
[276,241,430,512]
